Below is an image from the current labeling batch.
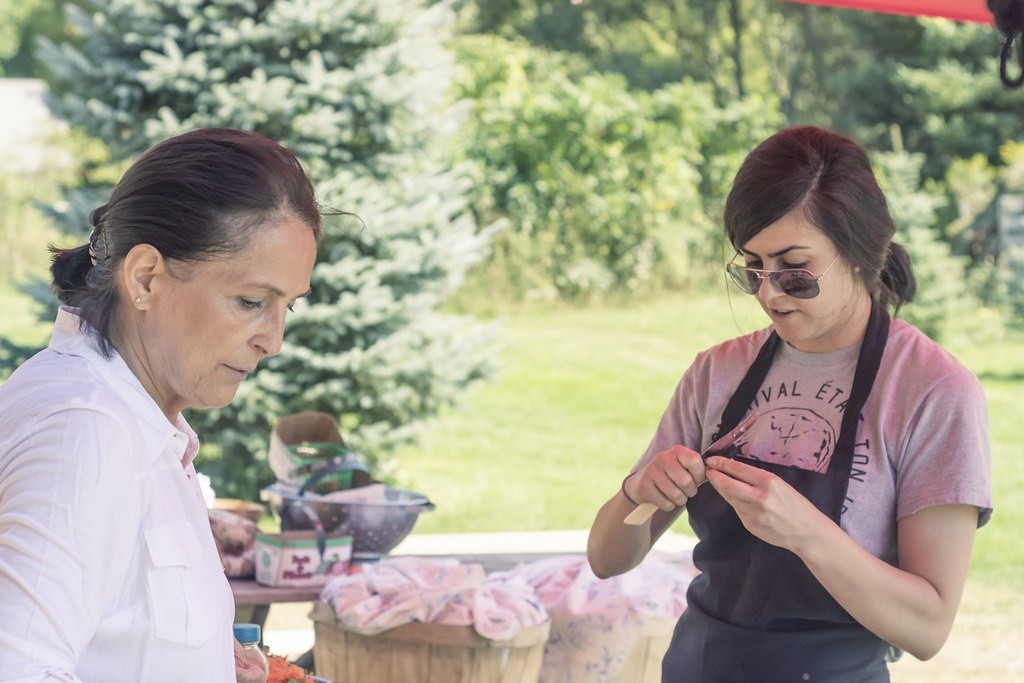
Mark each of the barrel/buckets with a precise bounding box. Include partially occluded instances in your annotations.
[309,604,552,683]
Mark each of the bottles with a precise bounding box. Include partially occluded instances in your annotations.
[235,624,269,682]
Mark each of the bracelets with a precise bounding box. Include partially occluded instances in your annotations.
[621,472,639,509]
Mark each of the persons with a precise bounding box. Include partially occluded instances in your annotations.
[0,128,321,683]
[587,127,994,683]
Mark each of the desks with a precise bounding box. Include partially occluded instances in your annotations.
[225,526,699,671]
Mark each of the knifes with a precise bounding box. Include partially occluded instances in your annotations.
[697,413,756,464]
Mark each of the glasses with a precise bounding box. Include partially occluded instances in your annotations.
[725,253,841,299]
